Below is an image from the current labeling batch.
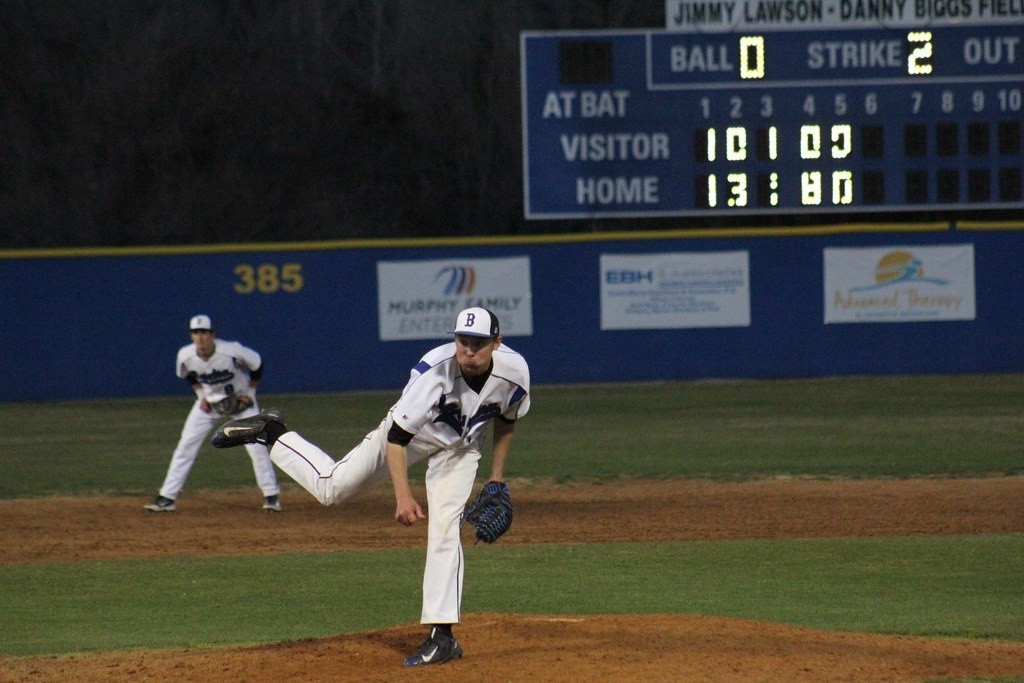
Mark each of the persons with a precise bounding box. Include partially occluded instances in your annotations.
[143,314,281,513]
[210,307,531,665]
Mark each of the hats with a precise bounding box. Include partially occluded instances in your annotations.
[190,314,211,331]
[447,306,499,337]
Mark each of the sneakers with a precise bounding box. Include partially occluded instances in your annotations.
[211,407,286,449]
[143,496,176,512]
[404,626,463,667]
[263,495,281,513]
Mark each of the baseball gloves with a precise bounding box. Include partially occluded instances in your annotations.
[214,394,254,416]
[464,480,514,544]
